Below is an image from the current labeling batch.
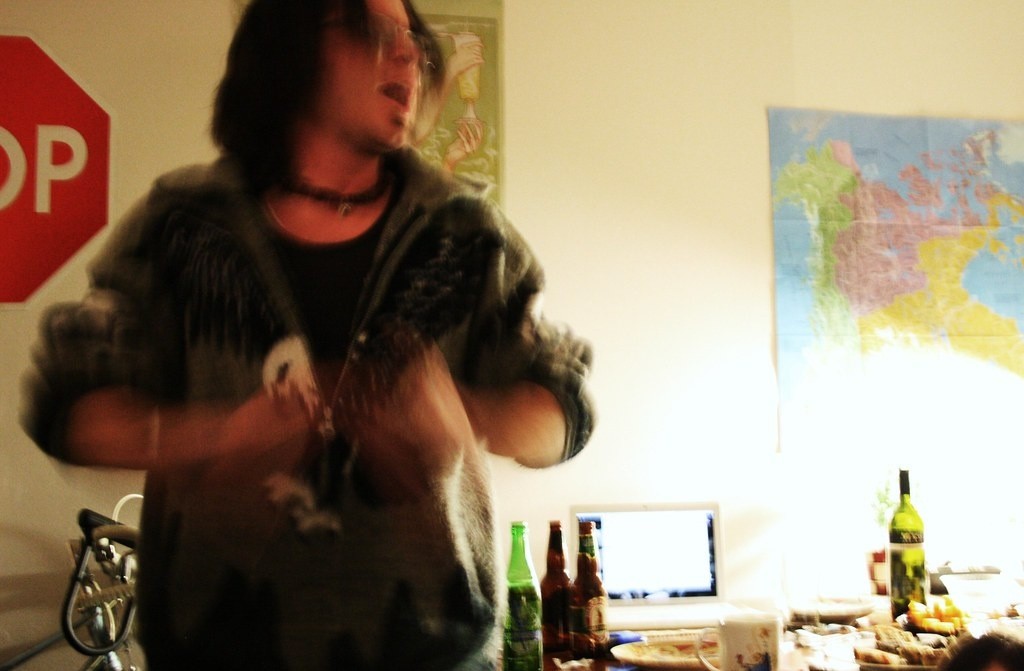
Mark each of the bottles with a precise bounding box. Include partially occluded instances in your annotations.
[569,521,609,659]
[505,521,543,671]
[889,470,924,623]
[540,520,577,662]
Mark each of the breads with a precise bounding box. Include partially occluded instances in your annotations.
[909,595,971,634]
[853,624,972,666]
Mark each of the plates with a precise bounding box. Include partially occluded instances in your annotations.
[611,636,730,669]
[790,598,874,627]
[857,659,940,669]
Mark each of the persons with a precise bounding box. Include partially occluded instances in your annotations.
[19,0,597,669]
[940,631,1024,671]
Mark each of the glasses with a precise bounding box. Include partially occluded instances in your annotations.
[320,10,432,68]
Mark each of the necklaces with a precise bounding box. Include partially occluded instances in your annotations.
[278,159,388,218]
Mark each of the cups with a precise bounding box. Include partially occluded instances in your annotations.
[695,614,784,671]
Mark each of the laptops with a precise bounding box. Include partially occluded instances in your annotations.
[568,501,767,632]
